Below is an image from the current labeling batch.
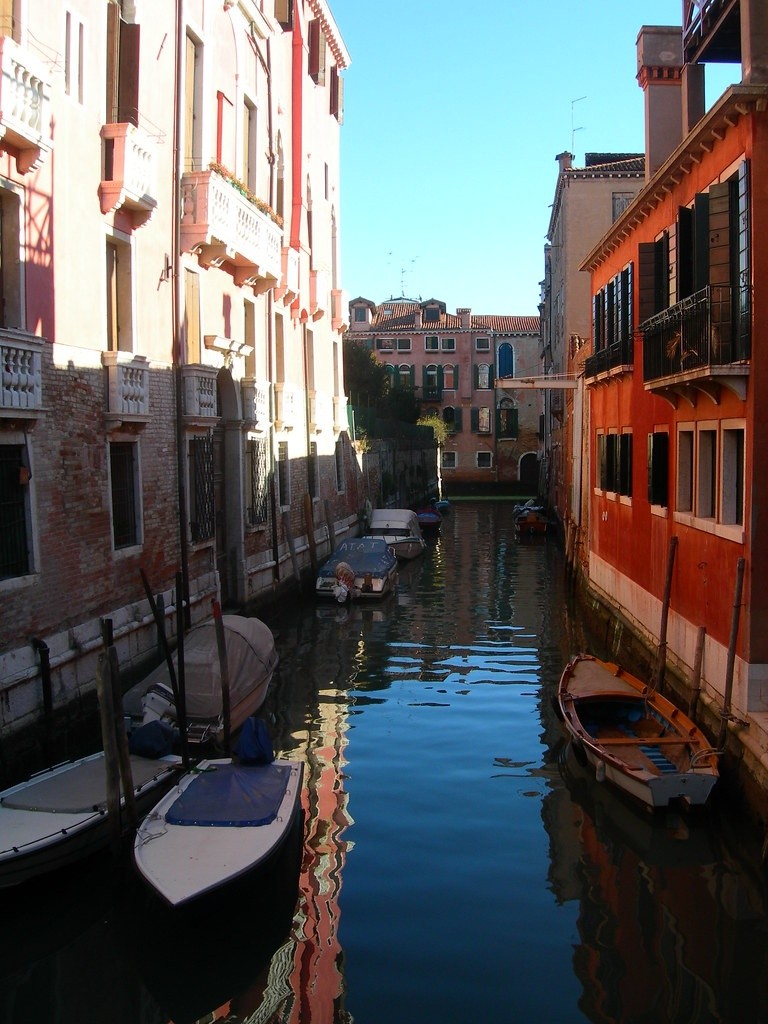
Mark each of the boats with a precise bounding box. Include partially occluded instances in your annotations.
[513,494,560,537]
[556,652,720,811]
[315,497,451,605]
[1,569,305,914]
